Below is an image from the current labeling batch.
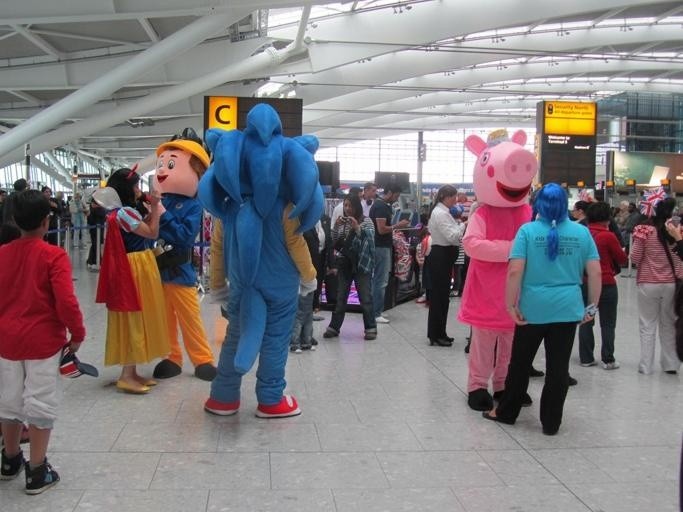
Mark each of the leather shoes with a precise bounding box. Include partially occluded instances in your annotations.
[143,378,157,386]
[116,376,150,393]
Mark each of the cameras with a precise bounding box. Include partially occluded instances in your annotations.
[340,215,352,224]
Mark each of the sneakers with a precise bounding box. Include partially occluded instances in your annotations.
[205,398,240,415]
[493,389,533,407]
[583,359,598,367]
[600,360,620,369]
[416,292,426,302]
[568,375,577,385]
[324,329,338,338]
[468,388,494,410]
[0,446,26,479]
[529,364,544,376]
[375,317,390,323]
[154,360,181,378]
[195,364,218,381]
[25,456,59,495]
[256,395,301,417]
[288,344,302,353]
[482,409,514,424]
[301,344,315,351]
[365,331,376,340]
[381,312,388,318]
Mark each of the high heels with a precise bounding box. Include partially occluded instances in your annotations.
[429,336,451,347]
[446,336,453,341]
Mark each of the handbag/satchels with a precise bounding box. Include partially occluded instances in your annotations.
[656,230,683,315]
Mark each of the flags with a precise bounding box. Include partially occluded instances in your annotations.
[579,190,593,202]
[640,187,666,218]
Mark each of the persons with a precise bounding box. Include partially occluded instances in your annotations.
[630,201,683,375]
[665,214,683,261]
[482,182,603,436]
[93,168,172,393]
[427,185,468,347]
[0,190,85,497]
[311,212,337,347]
[362,182,378,218]
[369,182,404,323]
[86,199,104,270]
[70,193,89,250]
[622,203,641,254]
[572,201,589,228]
[42,187,58,246]
[4,178,26,225]
[324,192,378,341]
[417,202,433,305]
[288,227,320,353]
[331,187,363,232]
[0,190,7,204]
[451,192,478,296]
[578,202,628,369]
[56,192,67,208]
[0,223,31,448]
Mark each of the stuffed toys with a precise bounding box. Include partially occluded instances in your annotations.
[149,127,218,381]
[457,129,537,412]
[199,104,326,418]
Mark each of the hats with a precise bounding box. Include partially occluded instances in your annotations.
[156,126,210,168]
[59,342,100,379]
